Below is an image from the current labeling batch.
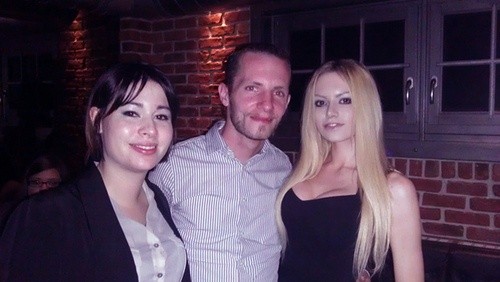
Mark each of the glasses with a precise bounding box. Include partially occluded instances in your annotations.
[28,179,61,188]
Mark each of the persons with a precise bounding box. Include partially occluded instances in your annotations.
[23,157,62,196]
[274,58,425,282]
[0,59,195,282]
[148,40,293,282]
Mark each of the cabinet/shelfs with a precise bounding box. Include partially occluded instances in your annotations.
[249,0,500,161]
